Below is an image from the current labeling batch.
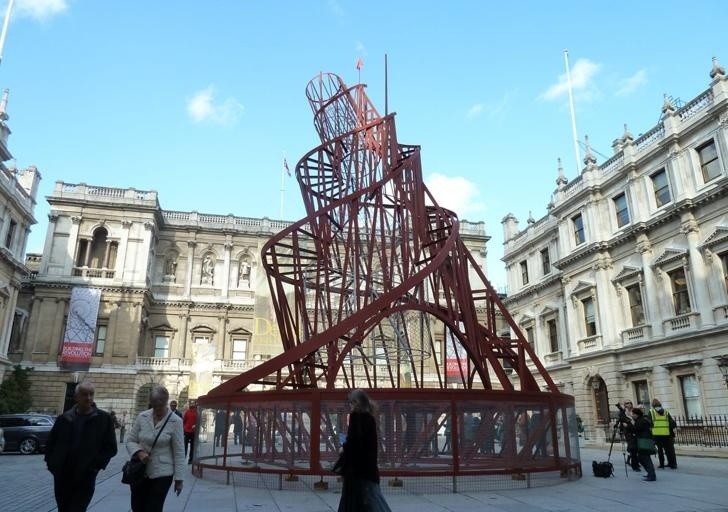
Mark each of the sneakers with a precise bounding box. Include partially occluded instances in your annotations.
[643,478,656,481]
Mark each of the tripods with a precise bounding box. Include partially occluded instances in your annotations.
[606,419,630,480]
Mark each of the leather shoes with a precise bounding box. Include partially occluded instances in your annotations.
[634,468,641,472]
[664,463,672,466]
[658,465,664,468]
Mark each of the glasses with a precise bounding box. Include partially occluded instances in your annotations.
[626,401,632,404]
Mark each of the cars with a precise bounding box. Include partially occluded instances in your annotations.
[0,427,5,455]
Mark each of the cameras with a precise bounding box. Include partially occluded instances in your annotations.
[614,403,627,417]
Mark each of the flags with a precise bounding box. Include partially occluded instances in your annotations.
[357,58,364,69]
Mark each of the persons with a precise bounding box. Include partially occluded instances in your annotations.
[202,255,214,277]
[165,256,176,276]
[622,399,656,482]
[442,408,555,454]
[118,409,130,444]
[232,411,243,445]
[43,381,117,512]
[238,257,251,279]
[648,398,678,470]
[109,410,121,429]
[170,400,182,418]
[329,388,393,512]
[179,399,198,465]
[119,385,186,512]
[212,409,227,448]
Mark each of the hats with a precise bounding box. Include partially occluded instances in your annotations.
[632,408,642,416]
[653,399,661,406]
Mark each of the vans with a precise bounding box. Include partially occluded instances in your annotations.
[0,413,58,455]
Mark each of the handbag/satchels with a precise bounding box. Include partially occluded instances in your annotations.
[638,439,656,452]
[593,460,612,478]
[122,456,147,484]
